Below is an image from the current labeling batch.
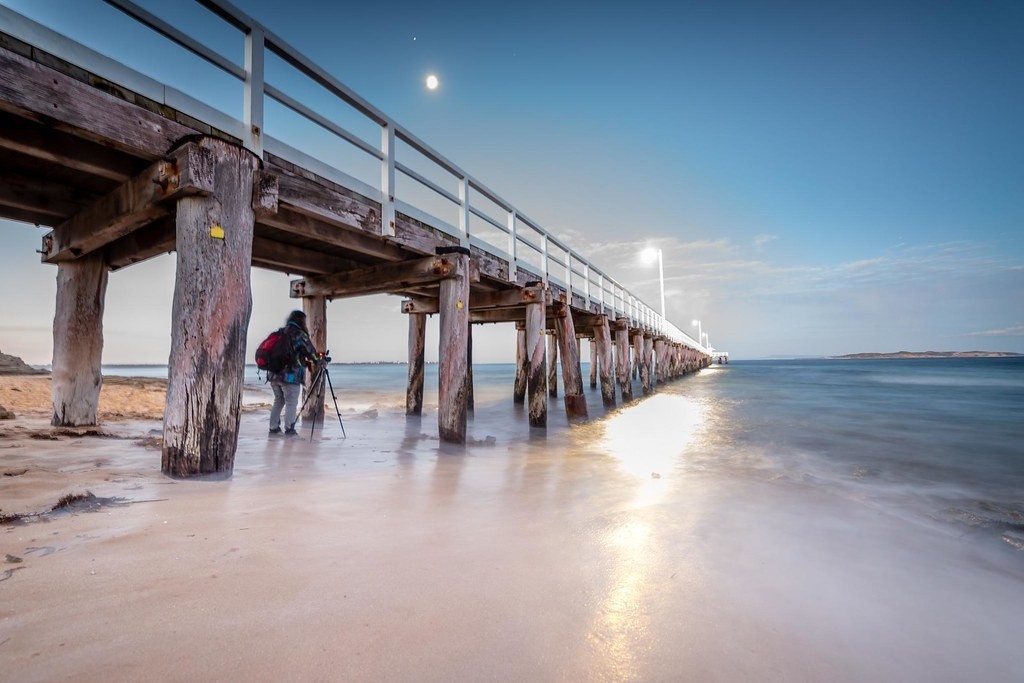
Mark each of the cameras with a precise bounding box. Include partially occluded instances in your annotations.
[318,350,333,365]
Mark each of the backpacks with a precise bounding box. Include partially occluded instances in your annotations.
[255,327,299,372]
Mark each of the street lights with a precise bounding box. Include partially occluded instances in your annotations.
[691,320,701,345]
[643,246,665,322]
[701,332,708,349]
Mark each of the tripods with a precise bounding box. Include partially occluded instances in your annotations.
[295,365,346,444]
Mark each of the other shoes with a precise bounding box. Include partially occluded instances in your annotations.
[286,429,296,433]
[270,427,281,433]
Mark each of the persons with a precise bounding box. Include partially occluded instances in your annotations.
[265,309,327,436]
